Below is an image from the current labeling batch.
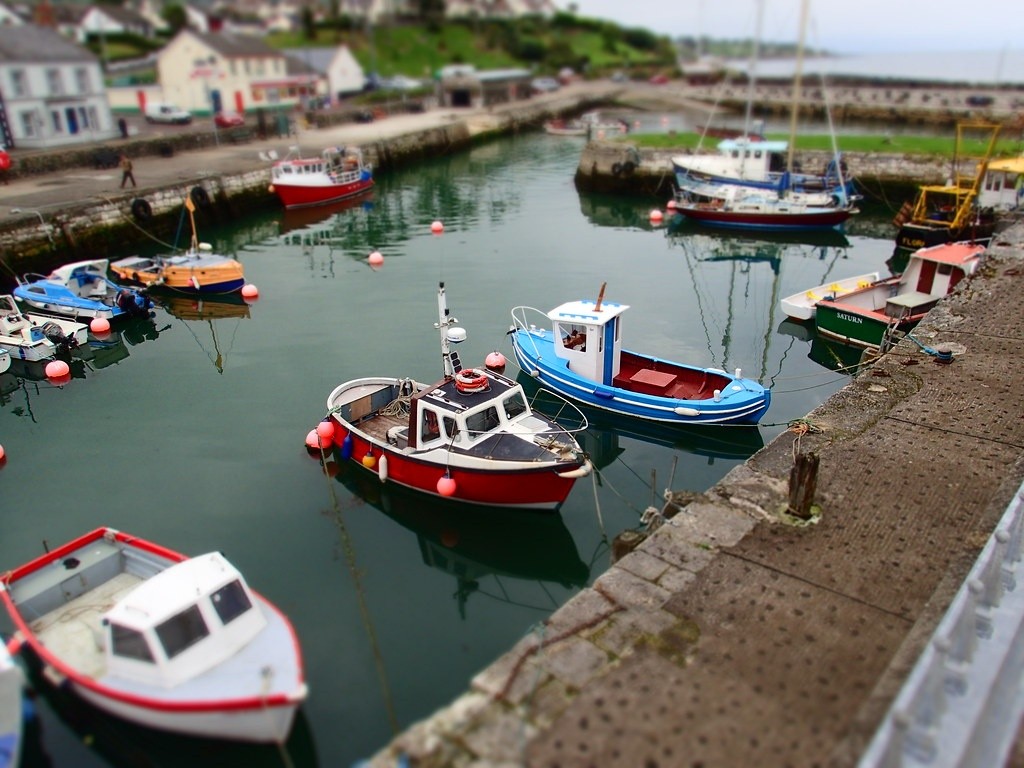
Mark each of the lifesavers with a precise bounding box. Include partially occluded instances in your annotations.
[131,198,152,221]
[190,186,211,210]
[455,369,489,393]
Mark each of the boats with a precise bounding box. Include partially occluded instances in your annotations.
[270,147,375,210]
[815,240,986,351]
[12,260,154,321]
[780,272,881,322]
[1,525,310,746]
[325,277,591,510]
[109,184,245,303]
[0,295,89,363]
[508,280,772,433]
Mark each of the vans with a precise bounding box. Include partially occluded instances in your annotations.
[145,103,191,124]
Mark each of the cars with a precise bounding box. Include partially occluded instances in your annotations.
[215,111,244,127]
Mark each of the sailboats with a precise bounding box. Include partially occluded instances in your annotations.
[672,1,861,235]
[674,1,865,204]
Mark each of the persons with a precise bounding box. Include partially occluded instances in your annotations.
[118,118,129,139]
[120,156,136,189]
